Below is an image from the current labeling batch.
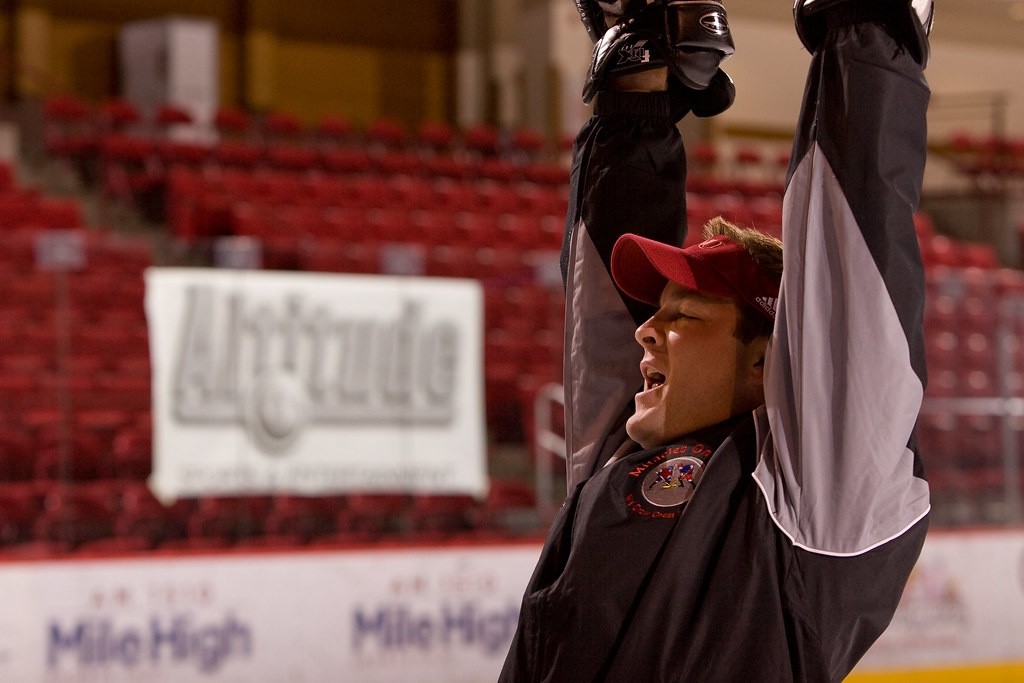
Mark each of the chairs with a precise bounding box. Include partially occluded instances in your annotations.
[0,96,1024,563]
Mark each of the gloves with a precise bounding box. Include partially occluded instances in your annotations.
[793,0,935,70]
[575,0,736,117]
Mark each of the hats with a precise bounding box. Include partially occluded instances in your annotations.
[610,233,778,322]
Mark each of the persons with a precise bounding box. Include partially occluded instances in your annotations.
[494,0,934,683]
